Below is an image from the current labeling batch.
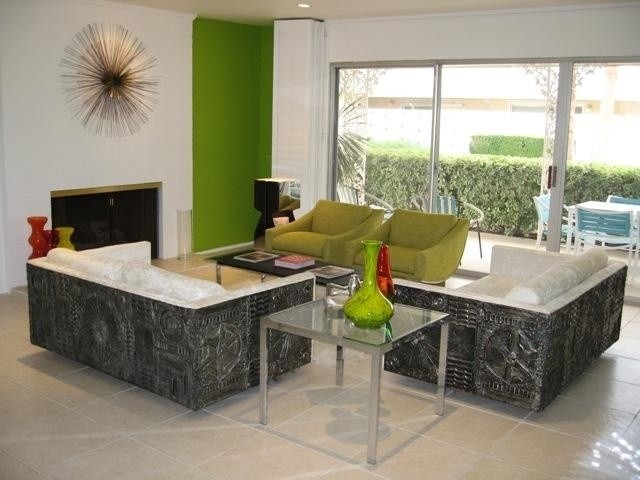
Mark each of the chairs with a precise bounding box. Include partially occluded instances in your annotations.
[602,194,639,255]
[573,205,639,271]
[412,196,484,266]
[532,194,575,253]
[336,182,394,224]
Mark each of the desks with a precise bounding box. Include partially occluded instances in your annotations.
[567,200,639,255]
[259,292,450,465]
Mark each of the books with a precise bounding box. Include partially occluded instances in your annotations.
[274,255,316,271]
[233,250,279,264]
[305,265,355,280]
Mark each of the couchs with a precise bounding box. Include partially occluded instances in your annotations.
[264,199,385,266]
[25,240,316,411]
[383,244,628,412]
[342,207,471,287]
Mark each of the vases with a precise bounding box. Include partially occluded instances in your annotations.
[27,216,49,259]
[56,227,75,250]
[44,230,58,255]
[342,239,394,330]
[376,243,396,339]
